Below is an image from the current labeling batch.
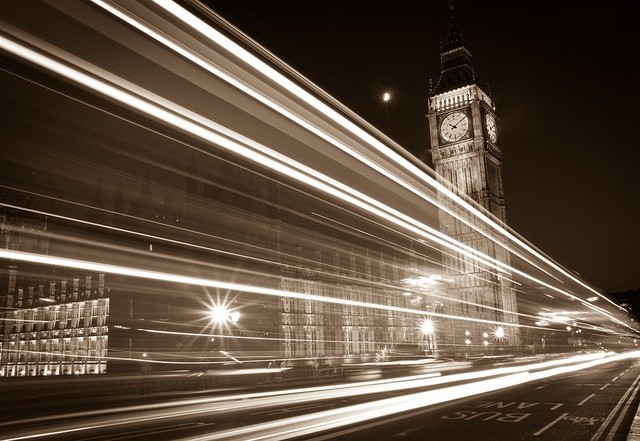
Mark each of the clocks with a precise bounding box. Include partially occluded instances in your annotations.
[484,112,498,144]
[439,112,469,143]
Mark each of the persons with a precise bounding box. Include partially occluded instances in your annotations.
[136,353,153,394]
[190,356,202,390]
[201,353,209,388]
[279,360,287,378]
[268,359,277,381]
